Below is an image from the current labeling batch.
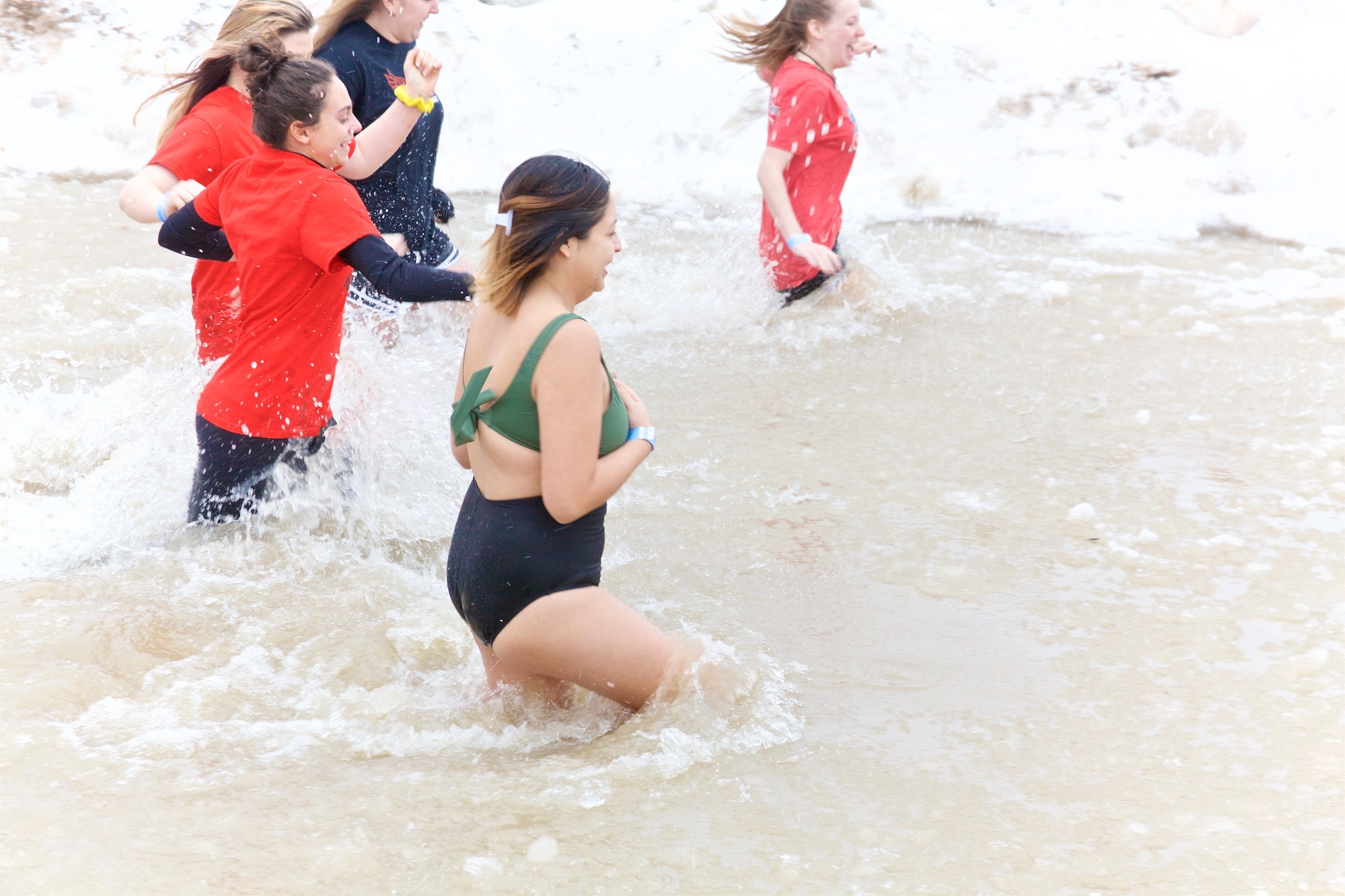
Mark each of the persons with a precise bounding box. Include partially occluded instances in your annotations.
[447,155,693,710]
[155,35,482,522]
[310,1,473,357]
[712,0,876,305]
[119,1,443,371]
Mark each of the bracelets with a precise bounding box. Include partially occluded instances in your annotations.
[157,203,168,222]
[786,233,811,250]
[393,85,434,114]
[626,425,656,450]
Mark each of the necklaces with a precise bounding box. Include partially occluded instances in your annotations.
[798,49,825,72]
